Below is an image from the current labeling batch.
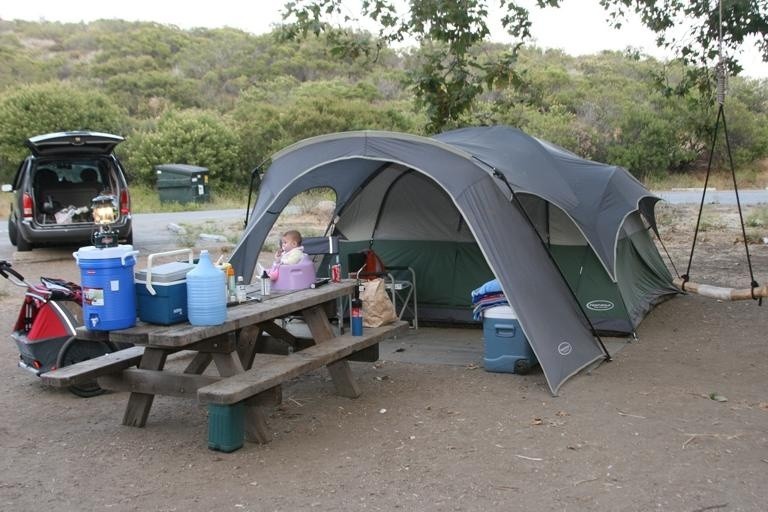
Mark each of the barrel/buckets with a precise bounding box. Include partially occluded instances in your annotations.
[71,242,137,333]
[186,249,228,326]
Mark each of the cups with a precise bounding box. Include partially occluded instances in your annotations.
[352,317,362,336]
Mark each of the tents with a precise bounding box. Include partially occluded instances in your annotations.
[206,119,685,395]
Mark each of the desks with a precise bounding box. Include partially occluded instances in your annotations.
[76,280,361,445]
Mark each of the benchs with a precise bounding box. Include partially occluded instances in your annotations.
[40,347,184,428]
[198,321,409,442]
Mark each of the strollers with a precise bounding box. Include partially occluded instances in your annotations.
[1,260,142,398]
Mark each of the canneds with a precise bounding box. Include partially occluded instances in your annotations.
[331,263,341,283]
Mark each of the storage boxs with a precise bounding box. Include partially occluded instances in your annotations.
[135,249,199,325]
[481,305,538,374]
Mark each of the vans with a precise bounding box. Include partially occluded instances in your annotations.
[8,129,134,252]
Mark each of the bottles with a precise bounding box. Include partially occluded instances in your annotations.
[227,268,247,305]
[332,264,341,282]
[261,271,271,296]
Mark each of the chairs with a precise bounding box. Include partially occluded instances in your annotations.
[348,251,418,340]
[280,237,345,335]
[35,169,103,208]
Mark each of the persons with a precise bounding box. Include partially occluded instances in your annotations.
[274,230,307,269]
